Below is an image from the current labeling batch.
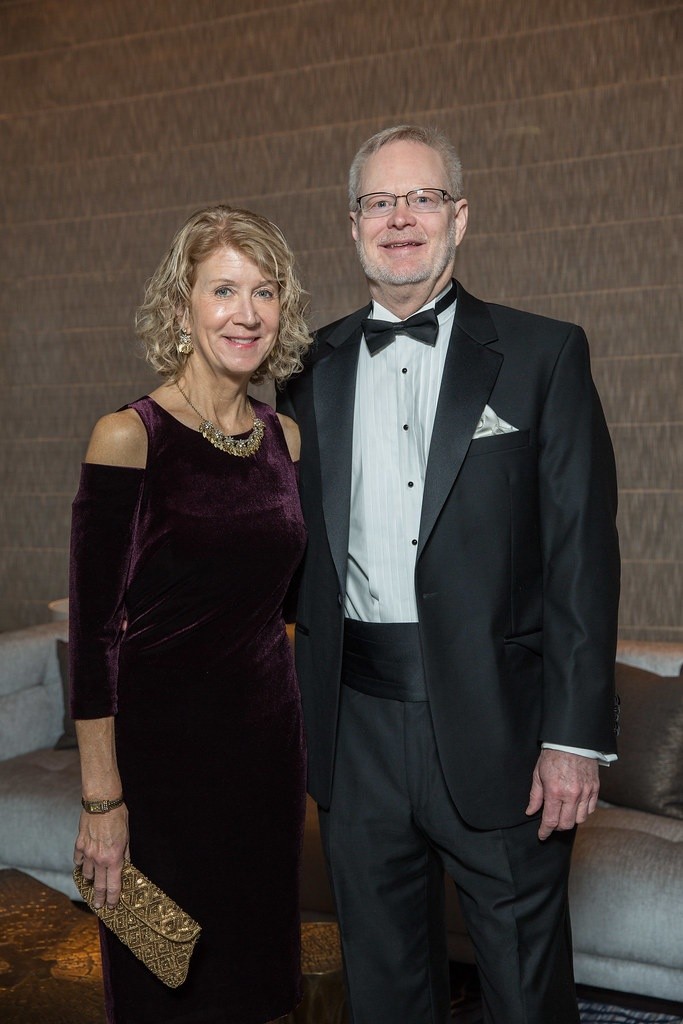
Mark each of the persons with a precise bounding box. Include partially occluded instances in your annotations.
[69,208,309,1024]
[120,124,621,1024]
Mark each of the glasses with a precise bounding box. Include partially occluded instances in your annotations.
[357,187,455,220]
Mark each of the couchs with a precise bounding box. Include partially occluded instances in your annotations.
[0,619,683,1017]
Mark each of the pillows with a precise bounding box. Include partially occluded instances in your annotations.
[55,639,79,751]
[597,661,683,820]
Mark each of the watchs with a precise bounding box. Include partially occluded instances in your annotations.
[80,796,124,814]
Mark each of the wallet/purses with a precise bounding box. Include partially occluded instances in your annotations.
[72,853,203,989]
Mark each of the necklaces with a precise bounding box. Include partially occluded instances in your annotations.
[174,379,266,458]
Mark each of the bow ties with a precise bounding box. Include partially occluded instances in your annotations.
[360,279,457,357]
